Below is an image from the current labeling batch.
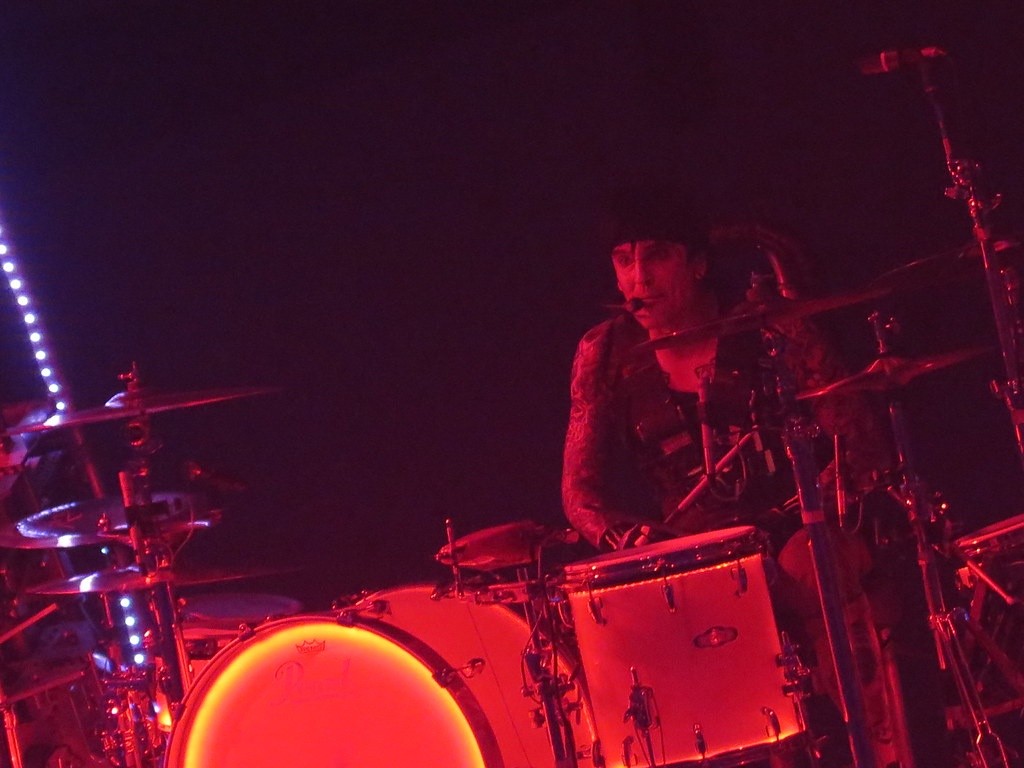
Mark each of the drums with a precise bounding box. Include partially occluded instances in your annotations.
[174,590,306,696]
[940,511,1024,731]
[563,524,812,760]
[170,583,595,767]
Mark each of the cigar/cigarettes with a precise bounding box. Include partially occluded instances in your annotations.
[623,296,662,312]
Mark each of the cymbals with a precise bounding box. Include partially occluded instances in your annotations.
[0,487,231,551]
[7,377,283,435]
[24,563,272,597]
[797,343,995,406]
[615,277,930,361]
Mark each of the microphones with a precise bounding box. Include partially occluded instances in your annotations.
[118,469,149,577]
[746,243,769,306]
[696,375,715,483]
[859,48,946,76]
[552,529,579,543]
[623,298,644,312]
[835,432,846,527]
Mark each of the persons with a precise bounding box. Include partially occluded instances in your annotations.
[559,205,966,768]
